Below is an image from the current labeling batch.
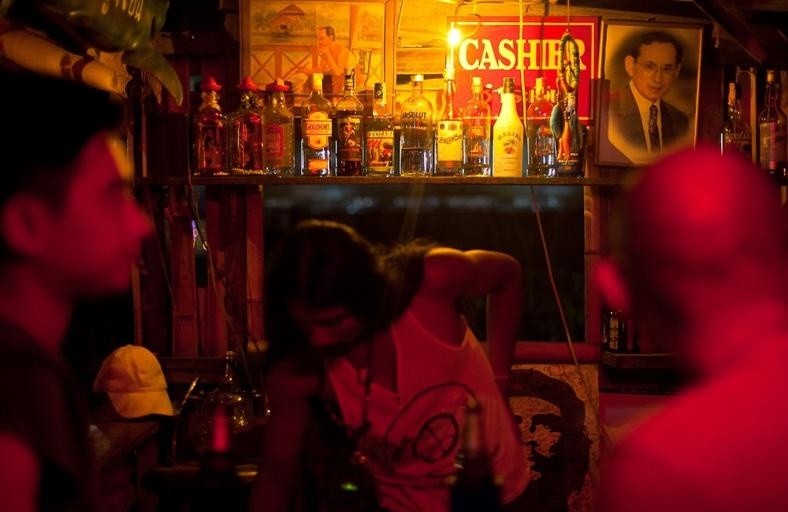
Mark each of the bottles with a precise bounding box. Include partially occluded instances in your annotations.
[398,75,435,177]
[364,82,394,174]
[494,75,524,177]
[300,73,335,174]
[198,350,253,460]
[436,69,462,176]
[527,75,584,179]
[192,78,297,177]
[337,68,364,175]
[464,78,491,175]
[725,71,786,177]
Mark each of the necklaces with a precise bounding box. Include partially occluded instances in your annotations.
[316,337,376,468]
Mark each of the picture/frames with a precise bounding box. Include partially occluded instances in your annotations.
[592,16,704,170]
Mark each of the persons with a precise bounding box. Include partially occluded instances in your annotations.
[316,25,366,95]
[344,121,353,132]
[566,145,787,511]
[611,29,693,154]
[248,216,543,511]
[0,72,159,510]
[548,34,589,171]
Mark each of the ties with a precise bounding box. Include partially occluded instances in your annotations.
[647,106,661,154]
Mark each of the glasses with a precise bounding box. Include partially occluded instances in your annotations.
[636,59,674,73]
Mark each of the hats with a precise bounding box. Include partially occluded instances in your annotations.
[92,344,176,419]
